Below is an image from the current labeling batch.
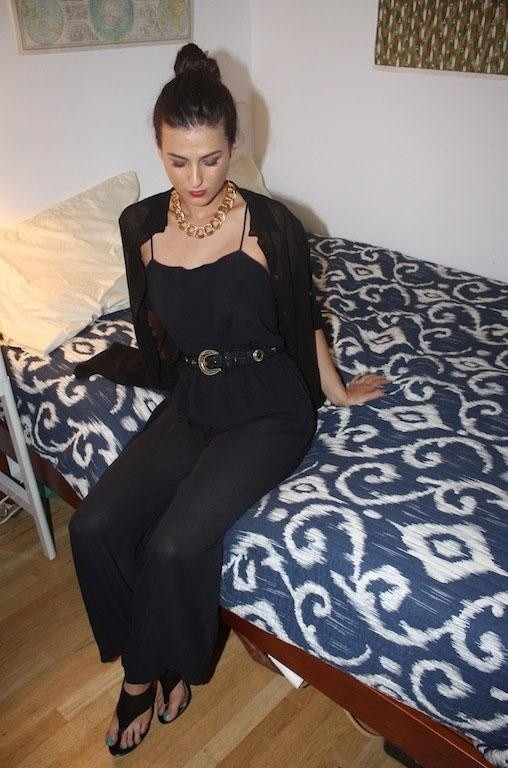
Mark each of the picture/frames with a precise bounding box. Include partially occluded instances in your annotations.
[8,0,195,56]
[372,0,508,81]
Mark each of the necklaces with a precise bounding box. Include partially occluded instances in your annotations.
[170,182,235,239]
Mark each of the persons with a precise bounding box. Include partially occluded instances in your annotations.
[68,42,391,757]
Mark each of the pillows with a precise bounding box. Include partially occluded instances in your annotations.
[226,156,272,197]
[2,170,140,356]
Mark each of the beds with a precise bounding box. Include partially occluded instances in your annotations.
[2,232,507,768]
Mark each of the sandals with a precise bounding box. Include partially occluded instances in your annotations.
[109,669,191,756]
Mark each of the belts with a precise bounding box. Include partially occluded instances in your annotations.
[184,344,282,376]
